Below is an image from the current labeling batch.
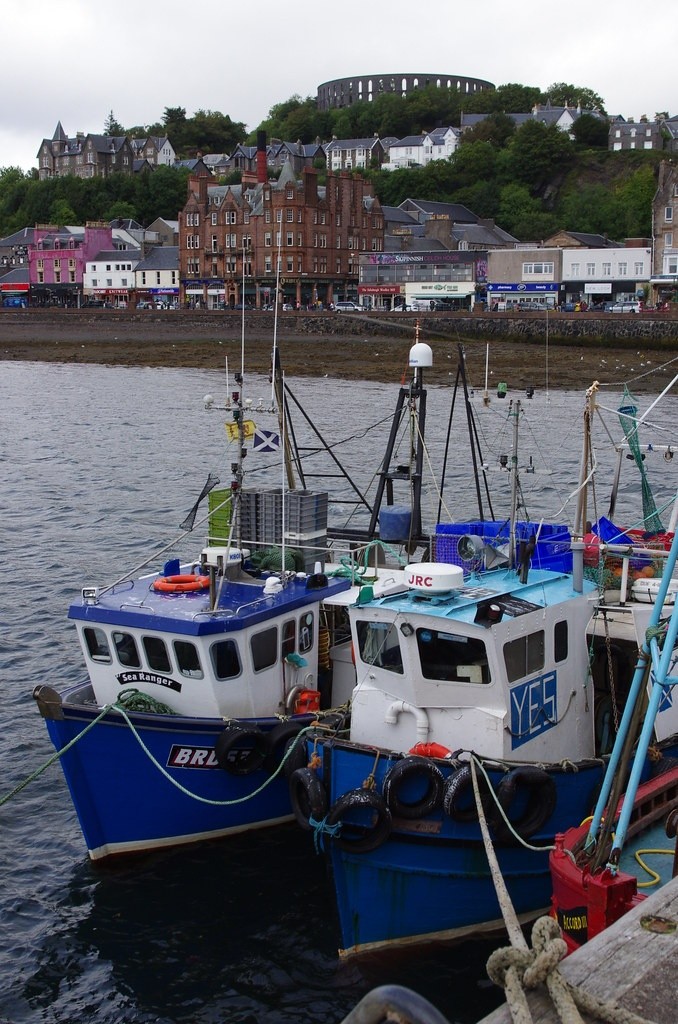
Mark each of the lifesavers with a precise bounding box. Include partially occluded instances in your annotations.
[266,721,306,779]
[588,771,644,817]
[649,756,678,780]
[152,574,211,594]
[327,788,394,854]
[380,755,448,819]
[487,766,557,846]
[441,764,496,824]
[215,722,270,776]
[288,767,328,833]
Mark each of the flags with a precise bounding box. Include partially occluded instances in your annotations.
[254,430,280,452]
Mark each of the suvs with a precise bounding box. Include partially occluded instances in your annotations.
[518,301,550,311]
[136,302,176,310]
[605,301,644,314]
[267,304,293,311]
[334,302,367,313]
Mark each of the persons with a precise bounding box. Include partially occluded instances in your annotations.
[297,301,301,310]
[196,300,199,309]
[330,301,335,310]
[156,299,169,309]
[402,303,406,311]
[575,301,588,311]
[430,302,435,311]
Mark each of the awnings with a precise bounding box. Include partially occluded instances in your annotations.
[240,288,304,294]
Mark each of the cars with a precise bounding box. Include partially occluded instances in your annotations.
[3,297,28,308]
[80,301,114,309]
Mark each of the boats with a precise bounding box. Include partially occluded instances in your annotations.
[32,209,578,862]
[288,354,678,970]
